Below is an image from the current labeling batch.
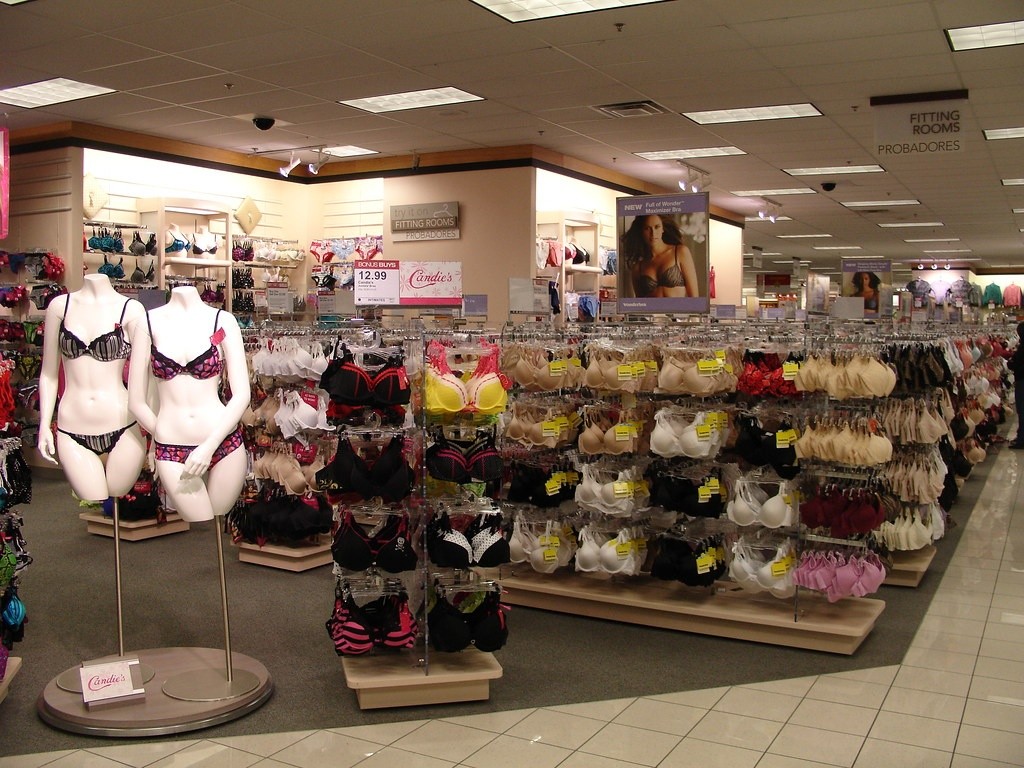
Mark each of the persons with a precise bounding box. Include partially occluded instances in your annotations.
[623,214,701,297]
[1006,298,1024,450]
[849,272,881,314]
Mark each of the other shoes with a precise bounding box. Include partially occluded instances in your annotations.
[1008,438,1024,449]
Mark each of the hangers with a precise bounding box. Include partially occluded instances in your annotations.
[0,221,1020,605]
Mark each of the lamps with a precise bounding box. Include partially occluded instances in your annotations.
[944,259,950,270]
[309,149,332,174]
[280,151,301,177]
[692,173,712,193]
[770,204,785,224]
[931,259,937,269]
[917,259,924,269]
[679,166,698,191]
[756,201,774,219]
